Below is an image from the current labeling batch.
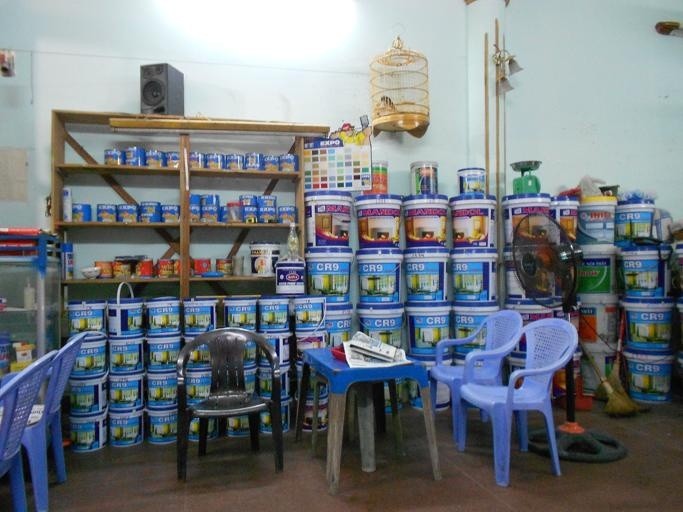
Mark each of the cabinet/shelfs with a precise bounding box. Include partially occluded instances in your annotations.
[1,233,63,403]
[49,106,308,353]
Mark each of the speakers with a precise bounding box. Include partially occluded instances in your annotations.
[140,63,184,116]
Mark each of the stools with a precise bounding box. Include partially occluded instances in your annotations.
[312,369,406,473]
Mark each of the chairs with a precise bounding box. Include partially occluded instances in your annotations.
[0,347,58,512]
[178,328,283,479]
[21,332,87,512]
[459,317,580,487]
[427,308,524,445]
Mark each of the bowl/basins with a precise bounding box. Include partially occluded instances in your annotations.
[81,267,101,280]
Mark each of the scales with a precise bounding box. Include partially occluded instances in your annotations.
[510,161,542,195]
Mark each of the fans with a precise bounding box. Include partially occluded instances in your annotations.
[512,212,628,463]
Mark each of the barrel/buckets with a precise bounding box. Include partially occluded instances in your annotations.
[58,282,681,451]
[74,203,92,222]
[105,148,180,169]
[365,160,390,196]
[305,245,673,303]
[189,192,297,223]
[412,161,439,195]
[95,254,180,277]
[455,167,487,196]
[191,256,244,276]
[304,190,660,247]
[96,201,180,223]
[248,239,281,278]
[192,150,298,172]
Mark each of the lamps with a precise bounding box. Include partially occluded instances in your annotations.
[108,117,330,139]
[493,41,525,95]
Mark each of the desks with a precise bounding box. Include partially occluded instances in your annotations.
[294,346,445,496]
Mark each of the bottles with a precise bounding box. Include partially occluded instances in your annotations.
[60,242,74,280]
[21,277,36,309]
[286,222,300,262]
[61,186,74,224]
